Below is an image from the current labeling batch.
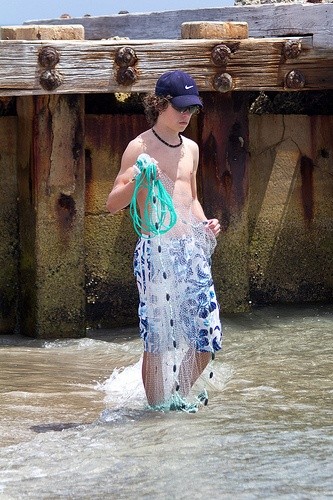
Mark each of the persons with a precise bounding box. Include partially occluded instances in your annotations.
[108,70,222,409]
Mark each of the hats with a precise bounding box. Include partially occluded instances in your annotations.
[155,70,204,111]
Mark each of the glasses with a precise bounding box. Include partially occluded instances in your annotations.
[169,101,199,114]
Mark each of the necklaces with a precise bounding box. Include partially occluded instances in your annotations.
[150,125,182,147]
